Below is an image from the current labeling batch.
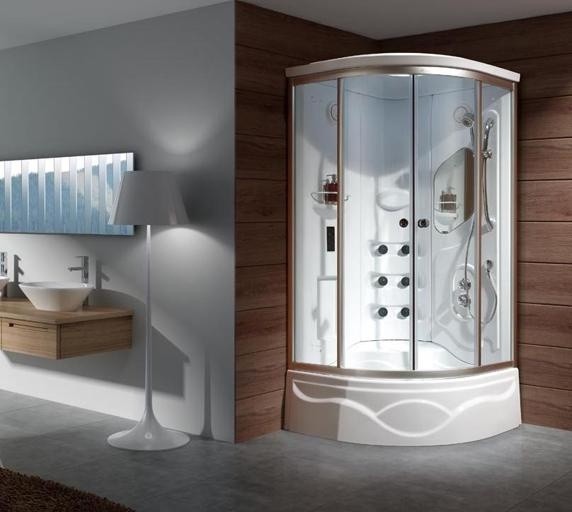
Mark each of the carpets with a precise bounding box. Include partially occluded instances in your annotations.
[0,466,136,512]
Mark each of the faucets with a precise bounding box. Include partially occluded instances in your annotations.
[66,255,89,311]
[0,252,9,297]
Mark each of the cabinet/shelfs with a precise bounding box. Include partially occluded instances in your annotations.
[0,315,132,360]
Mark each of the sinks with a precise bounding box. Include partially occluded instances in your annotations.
[0,276,10,292]
[17,281,96,311]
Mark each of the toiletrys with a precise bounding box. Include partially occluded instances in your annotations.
[439,184,456,213]
[322,173,339,204]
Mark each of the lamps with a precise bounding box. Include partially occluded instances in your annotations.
[105,168,192,452]
